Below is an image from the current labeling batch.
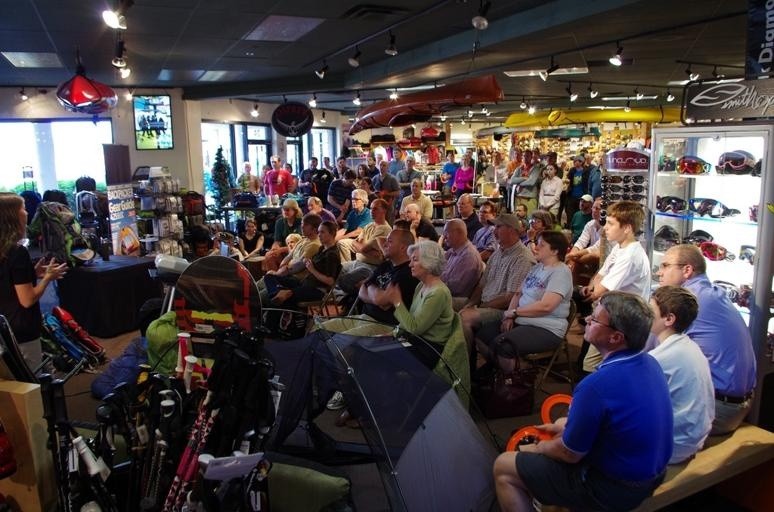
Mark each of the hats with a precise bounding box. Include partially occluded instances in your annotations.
[577,193,594,204]
[280,198,299,208]
[217,232,234,242]
[570,155,585,163]
[546,151,557,157]
[487,213,521,233]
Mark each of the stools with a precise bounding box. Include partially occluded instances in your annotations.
[633,421,774,512]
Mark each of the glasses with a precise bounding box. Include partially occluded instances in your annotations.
[688,197,741,218]
[660,262,688,269]
[600,211,607,216]
[601,194,622,200]
[478,211,492,215]
[739,245,756,265]
[635,230,646,236]
[700,242,727,261]
[689,230,713,242]
[676,155,711,175]
[640,203,648,208]
[599,218,606,226]
[622,194,647,201]
[351,198,361,201]
[718,152,755,171]
[656,196,689,214]
[370,205,384,210]
[600,201,616,209]
[623,175,648,184]
[622,185,648,193]
[591,311,619,331]
[600,175,623,183]
[601,184,623,192]
[655,224,681,245]
[529,218,542,224]
[456,201,471,206]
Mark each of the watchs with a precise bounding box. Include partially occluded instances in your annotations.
[527,239,535,243]
[512,307,518,316]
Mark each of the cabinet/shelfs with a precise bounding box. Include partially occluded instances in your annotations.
[646,125,773,426]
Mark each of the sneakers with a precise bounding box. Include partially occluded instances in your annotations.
[480,368,496,385]
[568,324,585,335]
[272,289,293,306]
[477,362,495,380]
[325,390,347,410]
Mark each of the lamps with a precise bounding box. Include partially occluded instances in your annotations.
[666,88,674,103]
[624,99,632,113]
[519,98,527,110]
[352,94,361,106]
[315,65,330,80]
[565,82,578,102]
[587,83,599,99]
[609,41,624,68]
[633,85,644,101]
[348,44,361,69]
[112,32,127,68]
[101,0,136,31]
[711,64,724,78]
[539,57,560,81]
[685,64,699,81]
[320,112,326,123]
[471,0,494,31]
[309,97,318,108]
[384,29,399,57]
[250,105,259,117]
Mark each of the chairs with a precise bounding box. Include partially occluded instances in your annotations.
[526,298,579,396]
[298,263,345,315]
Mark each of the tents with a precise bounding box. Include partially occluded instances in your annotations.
[255,314,498,512]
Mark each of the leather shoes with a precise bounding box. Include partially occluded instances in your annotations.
[334,410,353,426]
[343,418,363,429]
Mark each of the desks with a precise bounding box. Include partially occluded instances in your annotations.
[65,249,165,338]
[220,200,282,231]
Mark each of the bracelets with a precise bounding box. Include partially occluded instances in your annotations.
[394,302,402,308]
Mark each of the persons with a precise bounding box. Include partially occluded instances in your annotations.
[396,155,421,209]
[0,191,68,378]
[376,219,410,262]
[404,203,439,243]
[333,239,455,428]
[491,291,673,512]
[457,213,537,373]
[282,163,297,192]
[471,201,500,262]
[490,230,574,381]
[515,203,528,220]
[387,148,406,176]
[538,163,563,223]
[263,155,293,198]
[507,148,523,178]
[236,162,259,218]
[353,163,368,188]
[587,153,604,196]
[326,169,356,223]
[261,198,303,272]
[485,151,510,213]
[139,114,166,136]
[499,150,509,166]
[335,188,371,263]
[375,154,383,170]
[540,152,563,179]
[569,194,593,246]
[360,176,377,209]
[264,221,341,327]
[655,244,756,437]
[439,218,483,312]
[239,218,264,259]
[566,155,588,224]
[336,199,393,305]
[399,178,434,220]
[258,165,272,191]
[372,160,400,228]
[523,210,553,255]
[451,154,474,201]
[331,156,351,180]
[584,154,595,171]
[566,196,604,285]
[518,220,528,244]
[646,284,717,466]
[442,152,460,218]
[530,149,545,168]
[466,148,482,174]
[297,157,320,214]
[255,213,324,305]
[209,233,243,260]
[285,233,302,252]
[366,156,379,178]
[326,229,415,411]
[322,156,333,173]
[559,160,570,193]
[511,149,540,214]
[438,193,483,253]
[578,200,652,377]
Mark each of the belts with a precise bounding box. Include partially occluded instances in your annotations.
[451,291,468,297]
[715,389,755,404]
